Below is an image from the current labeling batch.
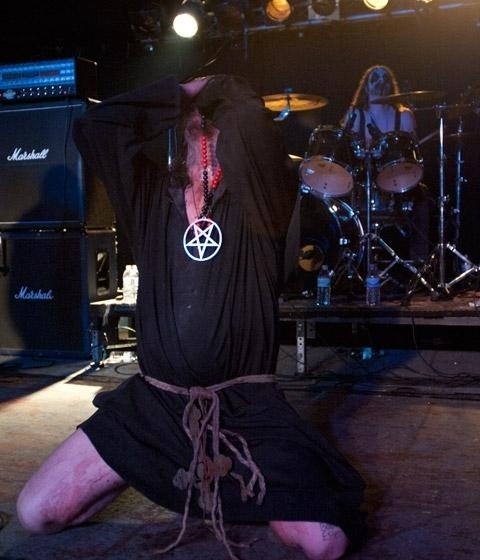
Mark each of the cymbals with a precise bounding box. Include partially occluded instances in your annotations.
[262,93,328,112]
[372,90,446,104]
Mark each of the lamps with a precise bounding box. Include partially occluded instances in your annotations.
[172,0,480,42]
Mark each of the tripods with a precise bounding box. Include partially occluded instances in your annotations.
[402,116,480,306]
[325,169,441,303]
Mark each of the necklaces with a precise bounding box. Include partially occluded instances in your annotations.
[190,178,200,219]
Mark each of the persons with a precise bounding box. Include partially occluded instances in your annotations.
[15,73,366,560]
[341,66,430,262]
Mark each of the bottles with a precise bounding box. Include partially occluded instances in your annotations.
[364,263,382,308]
[314,264,332,306]
[121,264,140,304]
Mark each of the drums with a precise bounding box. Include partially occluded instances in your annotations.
[299,186,366,288]
[298,123,356,196]
[370,130,424,193]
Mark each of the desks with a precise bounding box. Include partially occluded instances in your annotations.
[88,297,479,377]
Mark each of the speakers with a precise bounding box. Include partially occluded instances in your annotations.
[1,229,118,362]
[0,102,115,230]
